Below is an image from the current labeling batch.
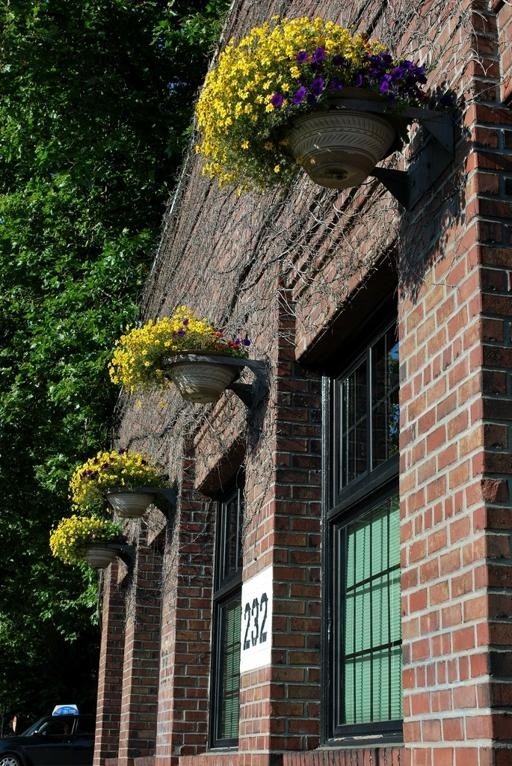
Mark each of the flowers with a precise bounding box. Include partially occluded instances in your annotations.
[107,306,250,409]
[194,15,427,199]
[49,449,170,570]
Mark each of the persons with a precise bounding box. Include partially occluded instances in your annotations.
[62,720,73,735]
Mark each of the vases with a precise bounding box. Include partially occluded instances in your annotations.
[162,354,245,404]
[275,86,404,190]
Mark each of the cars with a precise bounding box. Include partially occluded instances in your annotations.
[0,714,96,766]
